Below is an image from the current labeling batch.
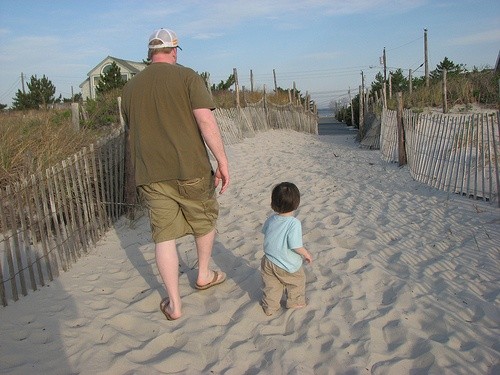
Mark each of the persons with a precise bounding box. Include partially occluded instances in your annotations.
[120,28,230,321]
[260,183,312,314]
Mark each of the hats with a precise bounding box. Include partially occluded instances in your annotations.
[148,27,183,50]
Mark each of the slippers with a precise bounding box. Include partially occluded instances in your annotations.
[160,297,178,320]
[195,270,226,289]
